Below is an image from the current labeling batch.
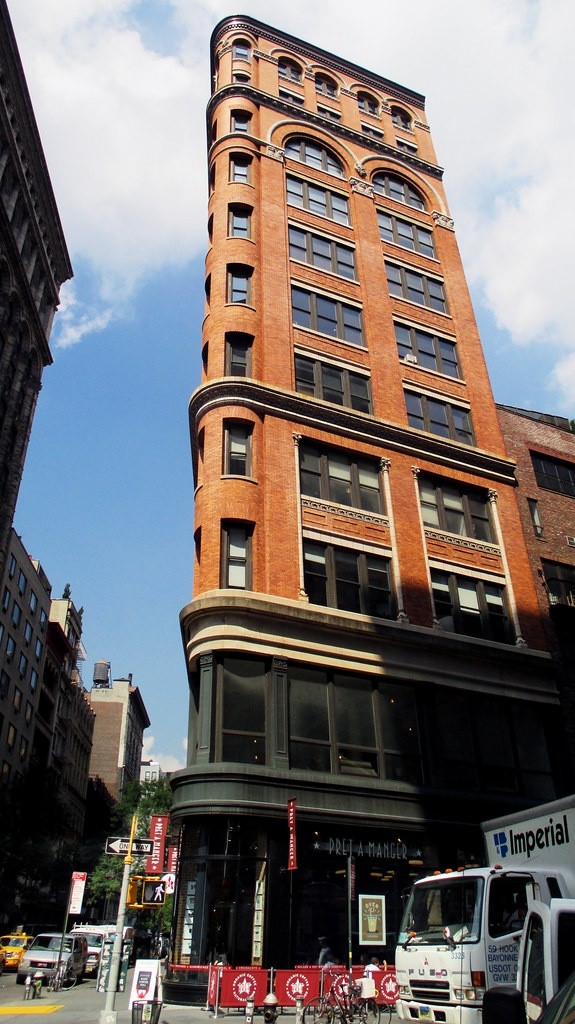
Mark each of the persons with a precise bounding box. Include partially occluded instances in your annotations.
[23,970,44,1000]
[363,957,381,979]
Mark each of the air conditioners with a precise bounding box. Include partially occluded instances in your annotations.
[404,354,417,364]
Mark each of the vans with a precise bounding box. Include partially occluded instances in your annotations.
[16,934,88,987]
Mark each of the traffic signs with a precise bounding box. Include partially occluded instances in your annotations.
[105,837,155,857]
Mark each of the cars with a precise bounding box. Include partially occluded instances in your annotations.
[0,935,34,967]
[0,944,7,975]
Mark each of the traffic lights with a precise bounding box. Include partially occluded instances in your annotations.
[142,879,166,906]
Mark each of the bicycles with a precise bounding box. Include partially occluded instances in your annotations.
[302,969,392,1024]
[48,960,77,992]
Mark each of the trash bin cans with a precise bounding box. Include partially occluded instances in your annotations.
[131,1000,163,1024]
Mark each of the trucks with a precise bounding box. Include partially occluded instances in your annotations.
[395,795,575,1024]
[70,925,133,974]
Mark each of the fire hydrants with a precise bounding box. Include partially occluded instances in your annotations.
[33,971,45,997]
[263,993,278,1024]
